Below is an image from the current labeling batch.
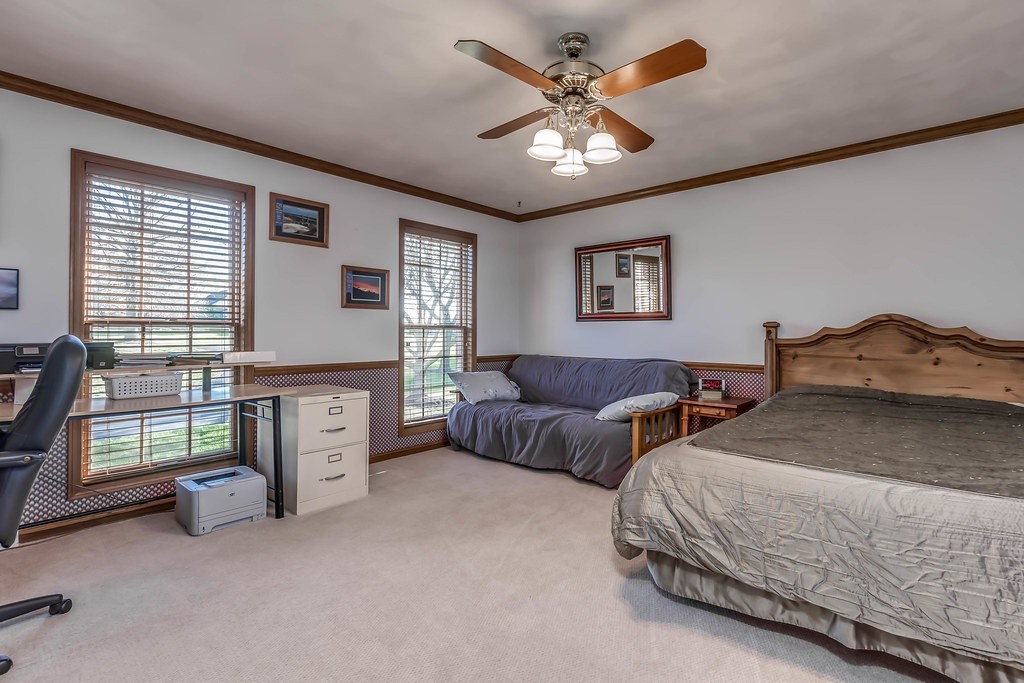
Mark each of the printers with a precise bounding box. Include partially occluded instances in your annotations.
[175,465,267,536]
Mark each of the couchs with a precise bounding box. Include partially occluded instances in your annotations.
[446,355,700,489]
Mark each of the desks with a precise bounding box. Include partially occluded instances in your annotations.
[0,383,297,518]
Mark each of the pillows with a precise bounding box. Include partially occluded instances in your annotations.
[594,391,679,421]
[447,370,520,404]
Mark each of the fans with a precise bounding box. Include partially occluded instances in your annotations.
[453,31,707,153]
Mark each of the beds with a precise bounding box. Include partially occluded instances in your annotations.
[612,314,1024,682]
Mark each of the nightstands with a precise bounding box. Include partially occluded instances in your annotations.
[677,396,755,436]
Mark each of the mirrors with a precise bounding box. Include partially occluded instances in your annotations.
[575,234,672,321]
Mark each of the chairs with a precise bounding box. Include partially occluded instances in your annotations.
[0,334,88,674]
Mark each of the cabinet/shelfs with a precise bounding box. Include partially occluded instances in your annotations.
[257,383,370,515]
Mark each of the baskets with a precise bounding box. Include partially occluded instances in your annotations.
[100,372,183,400]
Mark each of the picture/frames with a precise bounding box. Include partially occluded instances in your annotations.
[616,253,631,278]
[340,264,390,309]
[597,285,613,309]
[269,192,328,248]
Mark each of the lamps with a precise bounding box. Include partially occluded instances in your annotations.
[526,59,621,179]
